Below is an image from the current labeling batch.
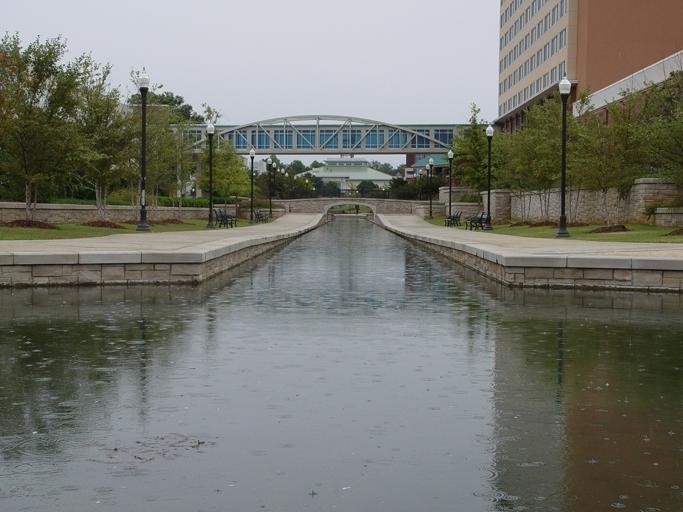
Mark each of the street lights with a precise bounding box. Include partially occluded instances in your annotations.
[206,123,214,228]
[419,169,422,200]
[557,72,571,237]
[267,158,315,218]
[448,150,453,216]
[248,149,255,224]
[484,125,494,229]
[425,158,433,216]
[136,67,150,231]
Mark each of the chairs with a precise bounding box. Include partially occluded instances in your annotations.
[213,207,238,229]
[443,210,462,227]
[253,209,269,223]
[465,211,485,231]
